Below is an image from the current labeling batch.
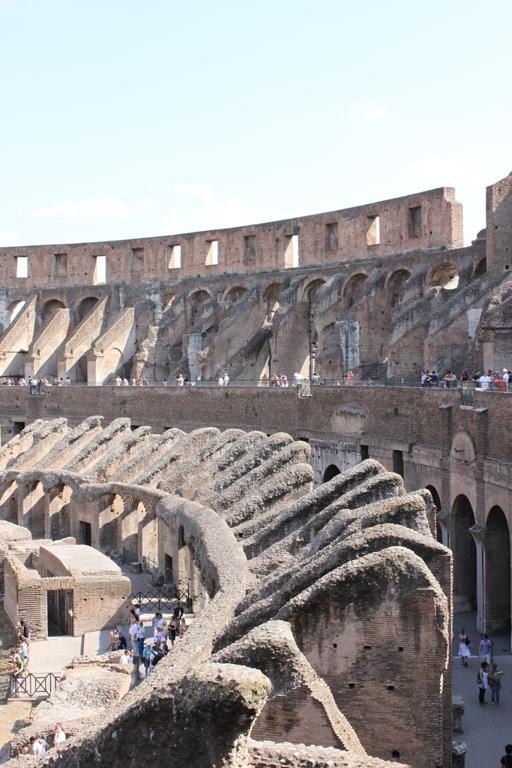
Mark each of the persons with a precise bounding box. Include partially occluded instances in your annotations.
[5,620,32,677]
[458,626,503,706]
[500,744,512,768]
[0,374,70,396]
[104,604,188,679]
[19,721,67,755]
[419,368,512,391]
[111,370,355,387]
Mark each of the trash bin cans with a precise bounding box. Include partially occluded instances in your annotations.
[110,549,124,566]
